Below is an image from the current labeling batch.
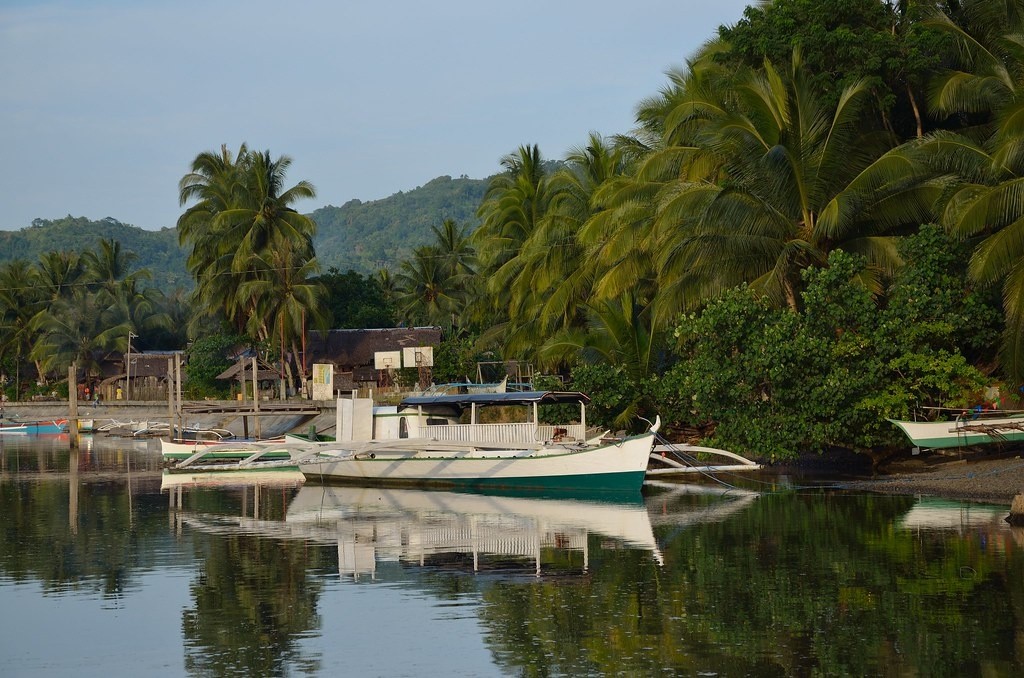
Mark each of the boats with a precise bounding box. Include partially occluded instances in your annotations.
[160,474,664,587]
[881,397,1023,453]
[288,366,664,486]
[158,424,335,464]
[0,420,67,433]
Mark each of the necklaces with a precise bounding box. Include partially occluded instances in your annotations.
[115,386,123,401]
[84,387,90,400]
[93,385,99,400]
